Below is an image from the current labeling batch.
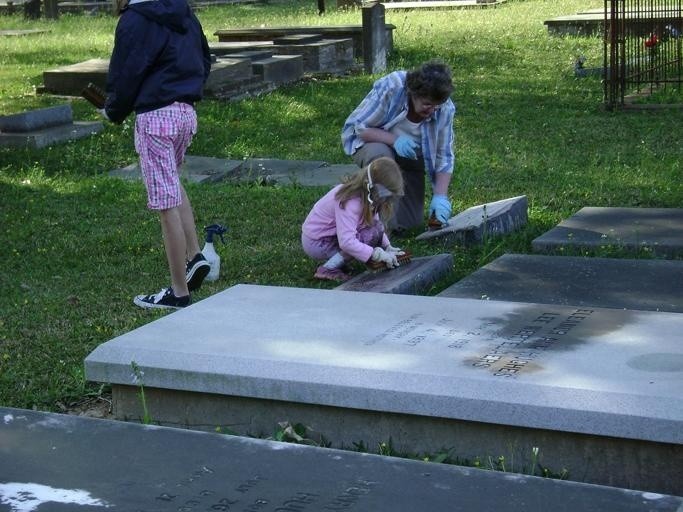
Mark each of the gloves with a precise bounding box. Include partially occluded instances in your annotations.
[428,194,452,224]
[394,135,419,161]
[371,247,401,269]
[384,245,401,254]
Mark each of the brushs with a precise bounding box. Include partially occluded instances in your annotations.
[81,83,110,110]
[428,213,443,230]
[368,251,413,274]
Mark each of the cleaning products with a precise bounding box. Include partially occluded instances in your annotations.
[200,223,227,282]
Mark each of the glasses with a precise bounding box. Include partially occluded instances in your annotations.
[416,96,445,111]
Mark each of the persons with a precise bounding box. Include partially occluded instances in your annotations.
[338,60,458,231]
[100,0,211,310]
[300,156,407,283]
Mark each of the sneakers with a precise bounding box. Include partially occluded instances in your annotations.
[313,266,352,282]
[185,253,211,294]
[133,287,190,310]
